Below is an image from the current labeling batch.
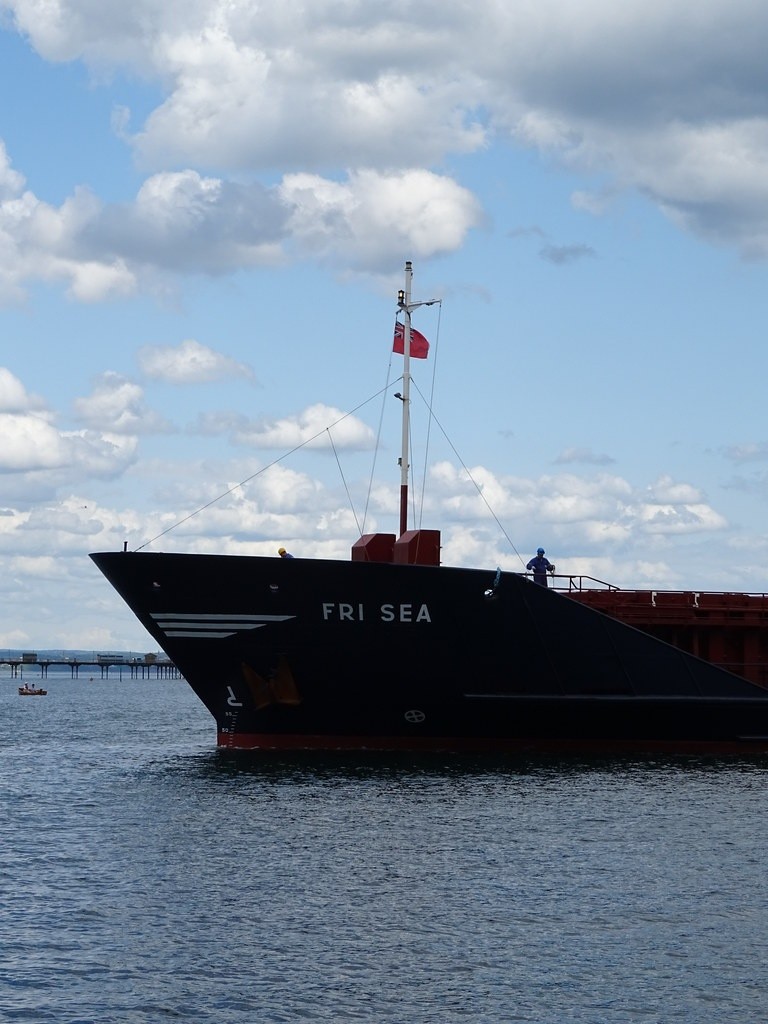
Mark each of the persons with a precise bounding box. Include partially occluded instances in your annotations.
[31,684,35,691]
[24,682,28,691]
[527,548,553,587]
[278,548,294,558]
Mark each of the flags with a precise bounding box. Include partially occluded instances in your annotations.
[392,320,430,359]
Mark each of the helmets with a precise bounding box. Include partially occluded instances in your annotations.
[537,548,545,554]
[278,548,286,554]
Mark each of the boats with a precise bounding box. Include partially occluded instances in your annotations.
[18,688,48,695]
[84,259,768,755]
